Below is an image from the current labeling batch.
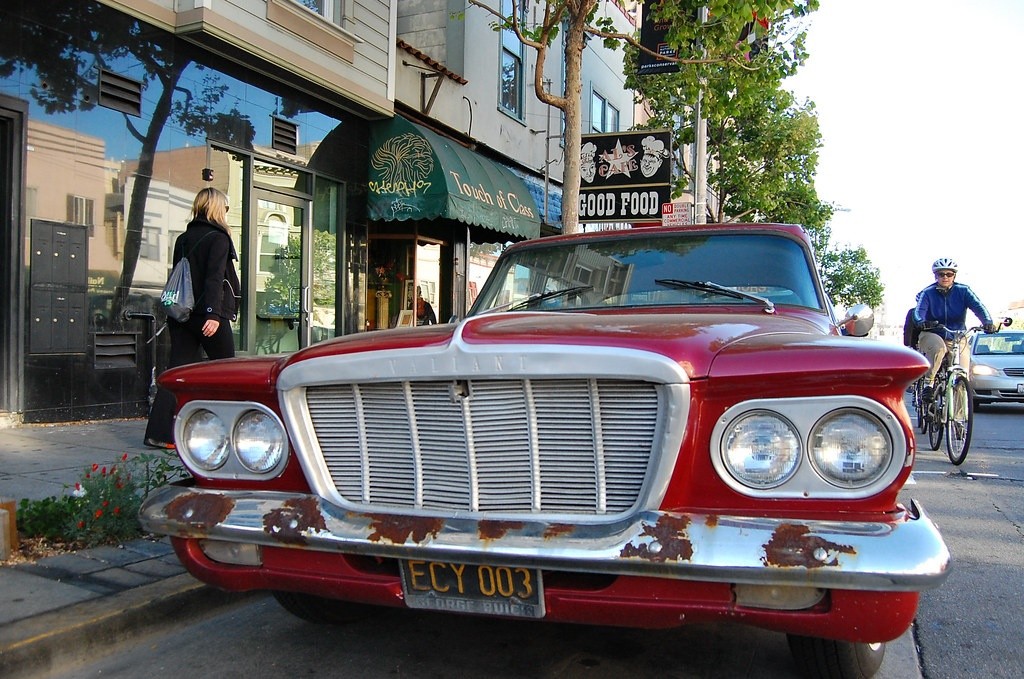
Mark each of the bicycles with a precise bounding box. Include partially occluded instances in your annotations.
[922,318,1013,467]
[905,345,930,434]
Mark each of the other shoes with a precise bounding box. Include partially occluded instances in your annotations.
[912,391,918,407]
[144,436,176,449]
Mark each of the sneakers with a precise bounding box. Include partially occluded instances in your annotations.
[956,426,965,440]
[922,385,935,402]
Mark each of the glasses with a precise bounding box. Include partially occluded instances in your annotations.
[225,206,229,213]
[938,272,955,278]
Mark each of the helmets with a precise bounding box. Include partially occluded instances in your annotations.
[916,291,924,302]
[932,258,957,273]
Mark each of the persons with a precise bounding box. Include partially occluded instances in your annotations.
[542,284,560,307]
[143,187,240,448]
[915,258,996,439]
[904,292,925,394]
[408,286,437,326]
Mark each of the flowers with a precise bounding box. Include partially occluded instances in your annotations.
[374,262,395,280]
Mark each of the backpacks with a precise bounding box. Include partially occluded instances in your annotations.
[161,230,218,323]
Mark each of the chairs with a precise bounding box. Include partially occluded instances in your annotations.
[1012,345,1020,351]
[977,345,989,352]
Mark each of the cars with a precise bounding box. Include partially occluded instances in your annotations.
[133,220,953,679]
[964,329,1024,411]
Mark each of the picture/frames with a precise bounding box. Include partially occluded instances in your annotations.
[404,280,414,310]
[396,310,413,327]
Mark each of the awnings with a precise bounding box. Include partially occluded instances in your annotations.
[294,123,361,239]
[369,114,541,243]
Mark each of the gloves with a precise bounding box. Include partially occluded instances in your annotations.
[984,323,996,334]
[918,321,931,330]
